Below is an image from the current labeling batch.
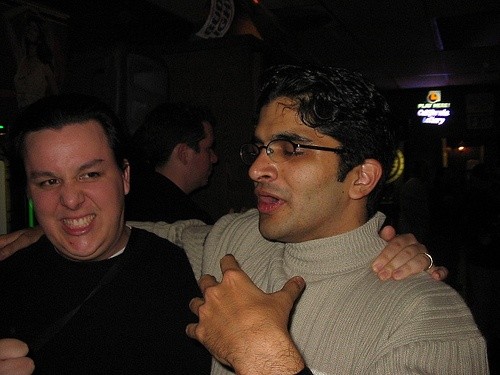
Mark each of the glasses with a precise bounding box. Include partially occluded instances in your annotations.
[240,138,343,165]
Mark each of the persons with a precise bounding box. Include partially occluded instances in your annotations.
[126,106,221,220]
[0,91,451,374]
[0,64,488,375]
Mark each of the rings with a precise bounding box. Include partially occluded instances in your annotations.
[422,252,433,270]
